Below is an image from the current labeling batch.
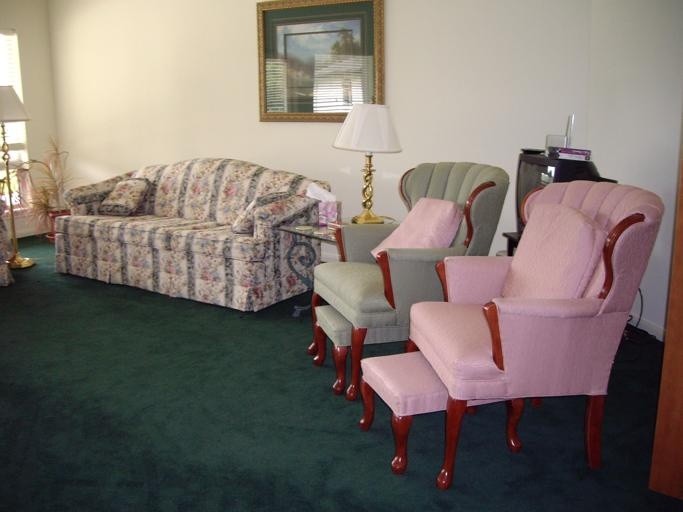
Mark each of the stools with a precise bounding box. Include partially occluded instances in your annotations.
[311,305,408,397]
[358,351,523,475]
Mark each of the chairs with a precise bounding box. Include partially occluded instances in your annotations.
[308,162,509,400]
[361,182,664,489]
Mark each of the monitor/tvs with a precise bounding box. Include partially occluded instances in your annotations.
[515,155,619,238]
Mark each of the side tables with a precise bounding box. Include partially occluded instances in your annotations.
[276,217,354,317]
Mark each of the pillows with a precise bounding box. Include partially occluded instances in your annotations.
[232,191,292,233]
[370,197,464,259]
[500,202,607,300]
[98,178,150,216]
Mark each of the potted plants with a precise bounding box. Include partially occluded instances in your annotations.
[22,138,71,245]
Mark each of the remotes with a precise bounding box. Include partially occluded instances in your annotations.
[521,147,545,155]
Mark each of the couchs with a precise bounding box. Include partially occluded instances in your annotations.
[56,158,340,311]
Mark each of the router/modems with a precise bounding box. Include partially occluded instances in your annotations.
[546,112,574,149]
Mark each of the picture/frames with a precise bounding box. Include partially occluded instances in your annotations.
[255,0,387,123]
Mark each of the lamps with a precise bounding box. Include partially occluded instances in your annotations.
[0,86,36,269]
[331,104,401,223]
